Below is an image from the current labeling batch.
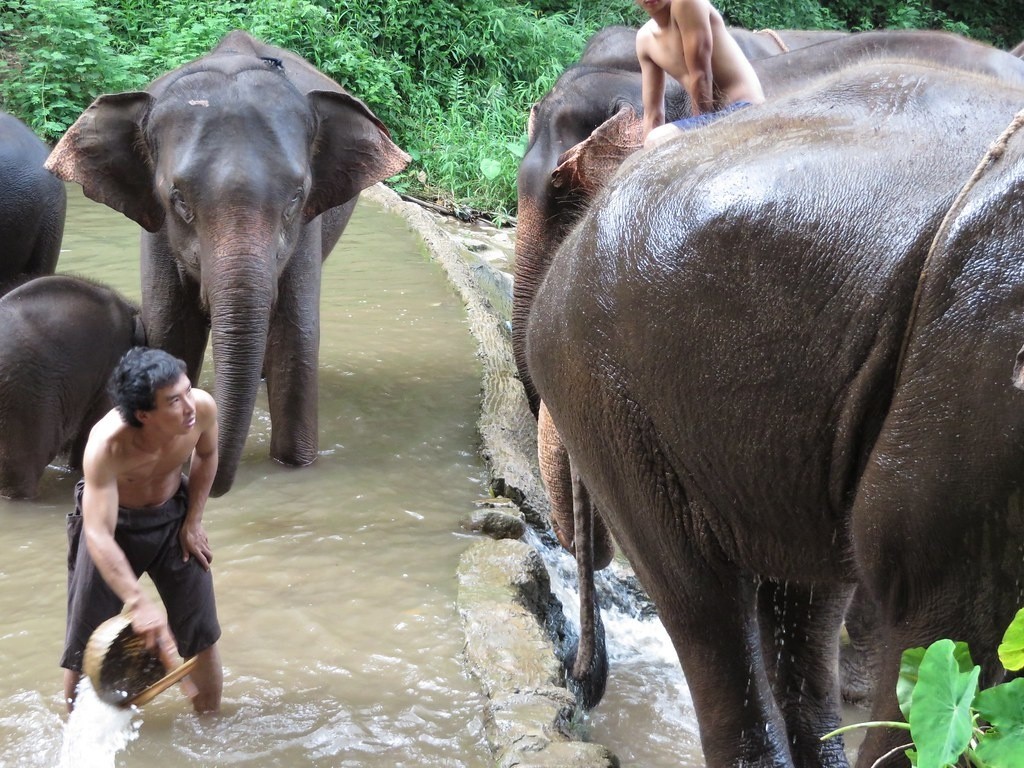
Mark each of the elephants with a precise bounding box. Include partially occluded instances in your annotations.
[0,110,138,493]
[42,31,412,498]
[512,25,1024,768]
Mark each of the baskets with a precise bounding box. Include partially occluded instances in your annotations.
[82,612,199,710]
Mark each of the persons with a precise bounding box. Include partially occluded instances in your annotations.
[610,0,766,178]
[58,347,223,711]
